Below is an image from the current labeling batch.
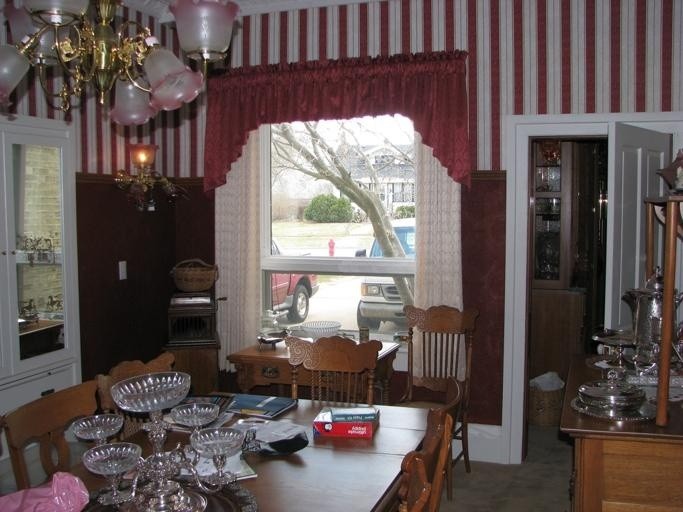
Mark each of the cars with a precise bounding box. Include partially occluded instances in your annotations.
[355,216,416,332]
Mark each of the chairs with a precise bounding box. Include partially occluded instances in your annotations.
[421,408,454,512]
[285,336,384,404]
[388,451,432,512]
[396,303,479,500]
[441,376,463,500]
[1,380,99,490]
[95,352,175,443]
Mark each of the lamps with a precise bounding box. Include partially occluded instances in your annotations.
[1,0,240,130]
[113,141,182,217]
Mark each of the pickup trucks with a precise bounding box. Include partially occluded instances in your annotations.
[271,237,320,321]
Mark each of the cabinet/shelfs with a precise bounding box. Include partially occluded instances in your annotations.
[1,112,81,460]
[527,138,581,288]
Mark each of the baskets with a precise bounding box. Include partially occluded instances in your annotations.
[172,258,218,291]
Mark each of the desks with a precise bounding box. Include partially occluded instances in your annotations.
[1,391,429,511]
[225,340,401,404]
[557,346,683,511]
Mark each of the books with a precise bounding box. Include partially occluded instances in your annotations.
[169,289,212,306]
[222,392,299,421]
[158,445,258,486]
[142,392,236,435]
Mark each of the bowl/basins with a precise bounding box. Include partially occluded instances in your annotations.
[301,321,342,341]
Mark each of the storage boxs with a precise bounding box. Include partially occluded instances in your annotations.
[312,406,379,441]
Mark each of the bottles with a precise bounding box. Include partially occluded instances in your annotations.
[360,326,369,344]
